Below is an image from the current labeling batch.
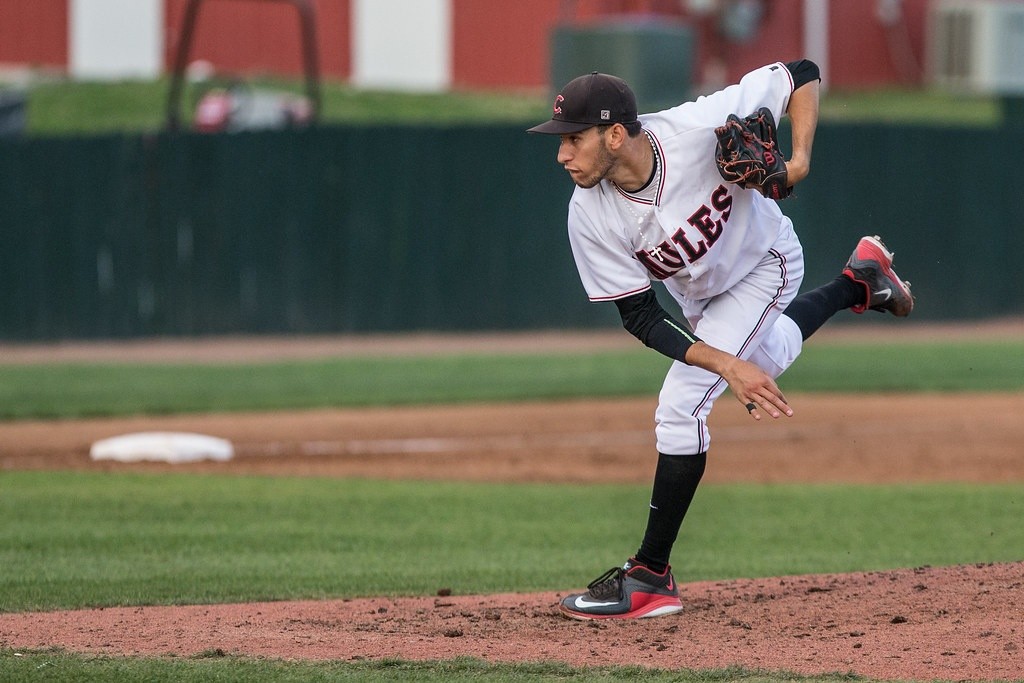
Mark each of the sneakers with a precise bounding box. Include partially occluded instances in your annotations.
[558,558,684,621]
[842,235,913,317]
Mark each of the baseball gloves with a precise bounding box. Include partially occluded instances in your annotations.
[713,107,794,200]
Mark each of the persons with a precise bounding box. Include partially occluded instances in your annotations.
[525,59,917,619]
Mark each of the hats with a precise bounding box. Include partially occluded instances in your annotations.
[526,71,638,134]
